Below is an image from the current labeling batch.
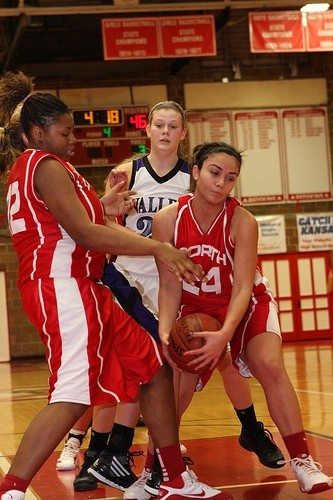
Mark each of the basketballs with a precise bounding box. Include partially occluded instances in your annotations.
[167,313,228,374]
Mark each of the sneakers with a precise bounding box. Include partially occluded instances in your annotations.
[157,469,234,500]
[0,490,25,500]
[144,468,164,496]
[87,449,146,491]
[55,439,81,470]
[123,465,152,500]
[239,423,286,468]
[74,449,101,489]
[277,452,333,495]
[145,432,187,454]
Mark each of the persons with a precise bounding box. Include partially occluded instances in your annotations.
[75,100,285,491]
[0,71,233,500]
[122,141,333,500]
